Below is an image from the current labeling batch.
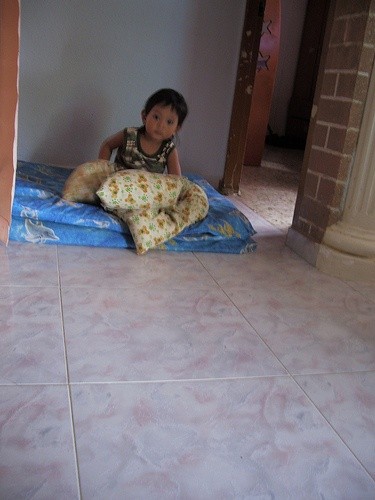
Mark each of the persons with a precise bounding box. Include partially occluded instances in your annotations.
[98,88,188,177]
